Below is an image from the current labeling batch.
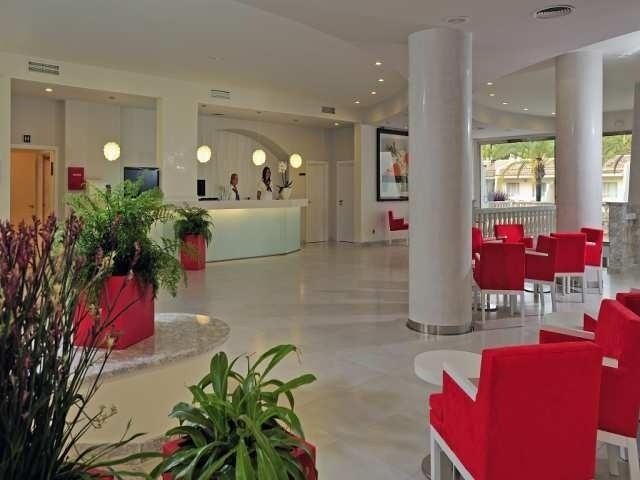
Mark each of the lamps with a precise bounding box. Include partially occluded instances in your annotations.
[252,111,266,167]
[197,104,212,164]
[103,96,121,161]
[289,119,302,169]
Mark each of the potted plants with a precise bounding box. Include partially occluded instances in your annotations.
[0,212,165,480]
[54,179,189,349]
[174,205,214,271]
[147,343,317,480]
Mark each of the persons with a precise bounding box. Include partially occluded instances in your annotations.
[222,172,241,201]
[257,166,274,200]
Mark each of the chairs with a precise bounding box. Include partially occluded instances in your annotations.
[428,342,602,479]
[385,211,409,246]
[537,290,639,479]
[470,224,604,327]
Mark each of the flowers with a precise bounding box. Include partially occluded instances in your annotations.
[275,160,297,192]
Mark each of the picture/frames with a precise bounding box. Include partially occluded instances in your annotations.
[376,128,409,202]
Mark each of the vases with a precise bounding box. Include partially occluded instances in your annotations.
[280,188,292,200]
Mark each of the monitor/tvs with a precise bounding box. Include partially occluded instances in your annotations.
[124,167,159,198]
